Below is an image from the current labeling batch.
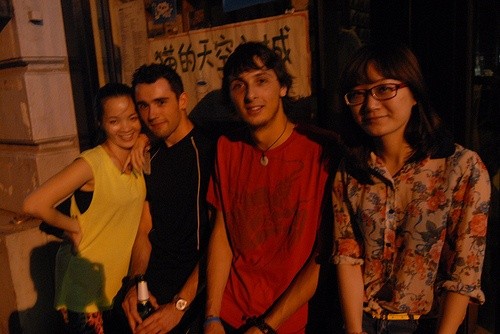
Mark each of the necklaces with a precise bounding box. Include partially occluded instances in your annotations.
[258,119,288,165]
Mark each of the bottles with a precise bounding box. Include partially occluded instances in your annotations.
[135,275,154,325]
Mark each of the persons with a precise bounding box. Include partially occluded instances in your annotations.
[23,82,149,334]
[202,41,334,334]
[330,43,491,334]
[121,64,215,334]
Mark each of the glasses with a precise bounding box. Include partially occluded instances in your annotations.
[343,80,409,106]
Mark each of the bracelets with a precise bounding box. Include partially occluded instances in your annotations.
[255,320,275,334]
[204,317,220,324]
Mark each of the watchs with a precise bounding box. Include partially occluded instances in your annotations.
[172,294,190,312]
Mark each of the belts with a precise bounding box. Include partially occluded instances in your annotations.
[370,309,422,322]
